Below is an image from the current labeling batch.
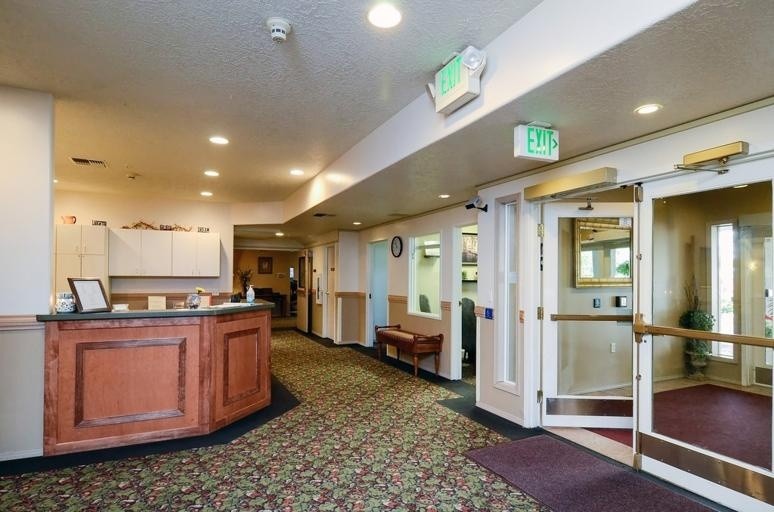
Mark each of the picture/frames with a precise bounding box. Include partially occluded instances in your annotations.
[67,277,112,314]
[462,232,477,266]
[258,256,272,274]
[462,264,477,282]
[299,256,305,288]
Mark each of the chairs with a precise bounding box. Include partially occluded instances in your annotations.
[462,298,475,376]
[419,294,430,312]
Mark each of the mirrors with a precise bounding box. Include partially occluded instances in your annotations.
[573,218,632,289]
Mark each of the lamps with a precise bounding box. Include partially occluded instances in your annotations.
[426,46,484,101]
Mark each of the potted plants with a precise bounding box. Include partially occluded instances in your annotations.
[679,310,715,380]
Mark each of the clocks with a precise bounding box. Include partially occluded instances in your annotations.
[390,236,403,257]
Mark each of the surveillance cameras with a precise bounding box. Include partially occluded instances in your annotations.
[465,196,481,210]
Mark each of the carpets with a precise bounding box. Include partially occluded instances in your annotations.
[462,432,718,512]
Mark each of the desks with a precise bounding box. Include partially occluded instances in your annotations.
[374,323,443,377]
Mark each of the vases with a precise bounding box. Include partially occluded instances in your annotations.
[241,283,247,298]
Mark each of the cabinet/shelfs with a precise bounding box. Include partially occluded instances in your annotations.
[109,228,220,278]
[52,224,109,309]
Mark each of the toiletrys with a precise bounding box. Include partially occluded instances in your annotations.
[246,285,256,303]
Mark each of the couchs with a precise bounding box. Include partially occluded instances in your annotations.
[253,288,287,318]
